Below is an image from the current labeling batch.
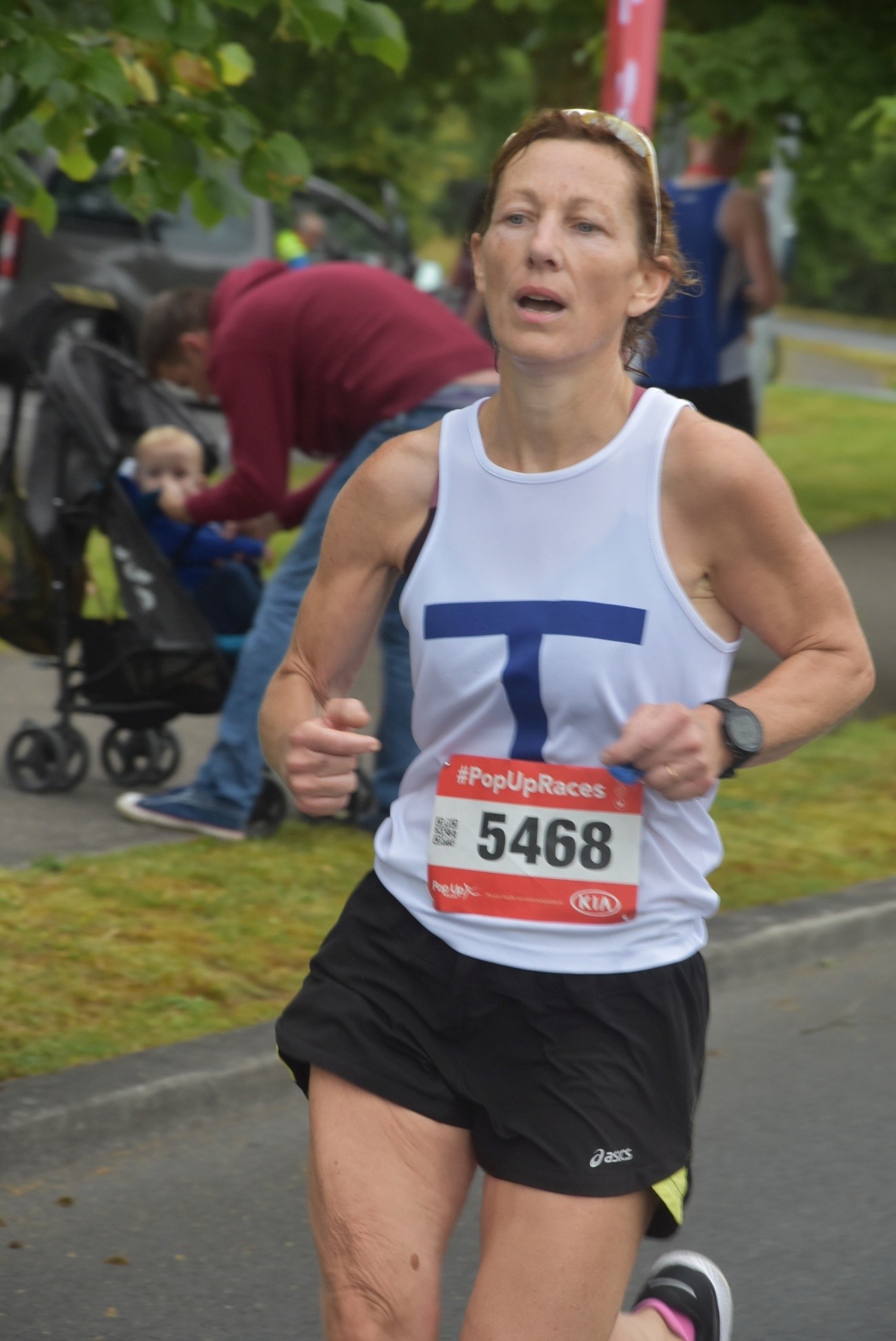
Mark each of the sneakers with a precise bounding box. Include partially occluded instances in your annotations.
[632,1251,733,1340]
[116,787,247,842]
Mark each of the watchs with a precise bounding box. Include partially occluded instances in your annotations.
[705,697,762,779]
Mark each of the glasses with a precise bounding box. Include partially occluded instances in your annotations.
[500,109,659,259]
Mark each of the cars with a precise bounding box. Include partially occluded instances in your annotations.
[1,134,415,393]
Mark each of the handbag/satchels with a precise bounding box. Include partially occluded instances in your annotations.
[0,543,77,656]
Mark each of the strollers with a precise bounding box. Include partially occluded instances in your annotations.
[1,266,265,796]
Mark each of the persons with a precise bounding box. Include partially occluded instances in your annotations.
[118,426,275,582]
[258,107,876,1341]
[273,207,325,268]
[635,101,796,436]
[116,259,502,839]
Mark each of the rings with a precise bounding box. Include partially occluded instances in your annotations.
[666,766,678,782]
